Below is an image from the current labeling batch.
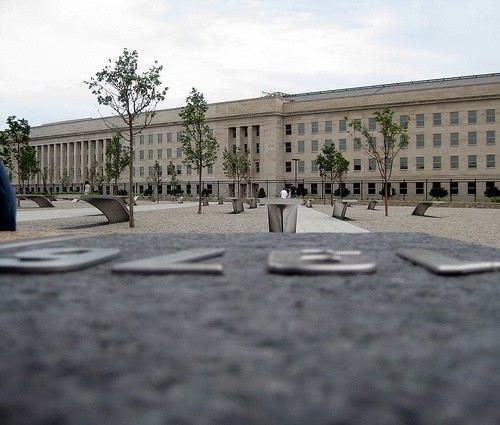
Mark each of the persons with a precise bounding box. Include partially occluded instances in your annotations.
[85,181,91,195]
[280,188,288,199]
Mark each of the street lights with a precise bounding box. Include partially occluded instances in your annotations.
[292,158,300,196]
